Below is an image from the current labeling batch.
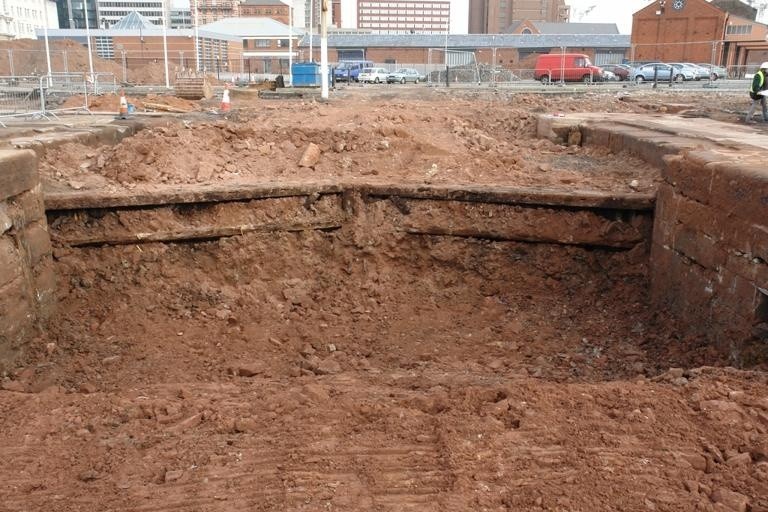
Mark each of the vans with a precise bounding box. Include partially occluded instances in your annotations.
[333,61,373,82]
[533,54,605,84]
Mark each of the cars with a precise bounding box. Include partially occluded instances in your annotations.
[632,62,711,85]
[385,68,421,84]
[599,63,636,81]
[699,64,727,82]
[357,67,391,84]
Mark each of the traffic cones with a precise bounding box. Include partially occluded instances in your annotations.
[229,73,241,88]
[114,88,135,121]
[250,72,256,84]
[219,82,232,113]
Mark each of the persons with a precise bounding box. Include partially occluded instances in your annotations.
[745,61,768,124]
